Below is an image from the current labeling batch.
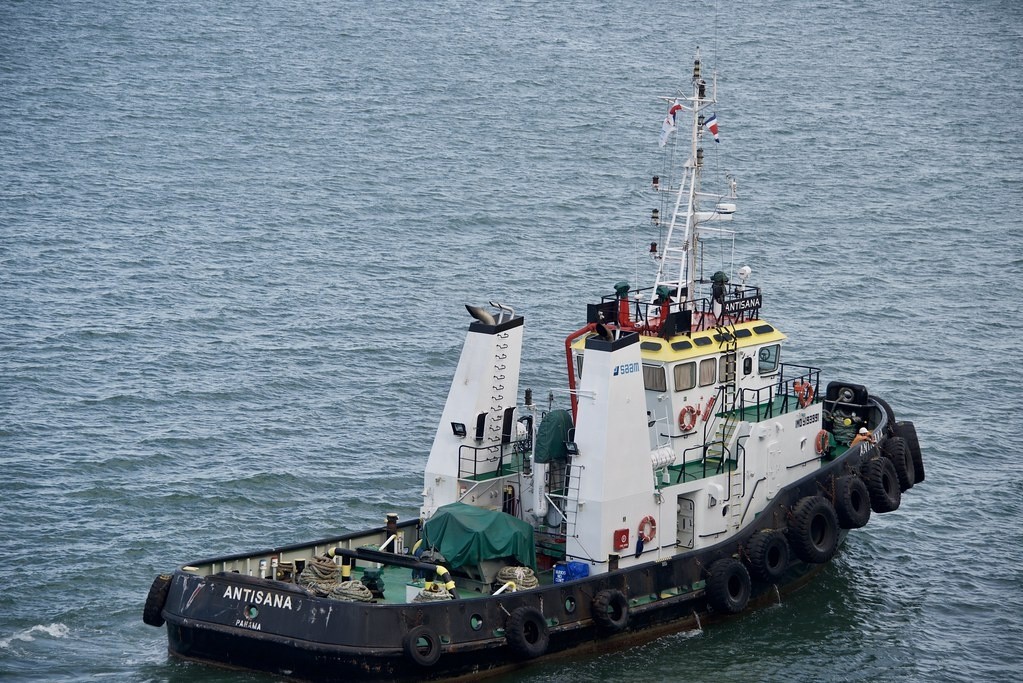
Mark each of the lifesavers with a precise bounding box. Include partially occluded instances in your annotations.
[868,394,925,482]
[708,558,750,610]
[404,625,442,665]
[750,530,790,579]
[862,458,901,512]
[638,517,656,542]
[679,405,696,430]
[814,430,829,453]
[144,573,174,624]
[790,496,837,561]
[508,609,550,655]
[833,477,870,529]
[592,589,629,627]
[881,436,915,491]
[799,381,813,408]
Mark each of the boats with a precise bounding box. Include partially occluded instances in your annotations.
[143,47,926,683]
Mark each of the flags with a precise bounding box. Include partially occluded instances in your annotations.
[704,115,720,143]
[669,99,682,127]
[659,118,676,147]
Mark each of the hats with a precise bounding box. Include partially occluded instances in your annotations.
[859,427,869,433]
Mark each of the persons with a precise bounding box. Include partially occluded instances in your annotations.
[850,428,874,448]
